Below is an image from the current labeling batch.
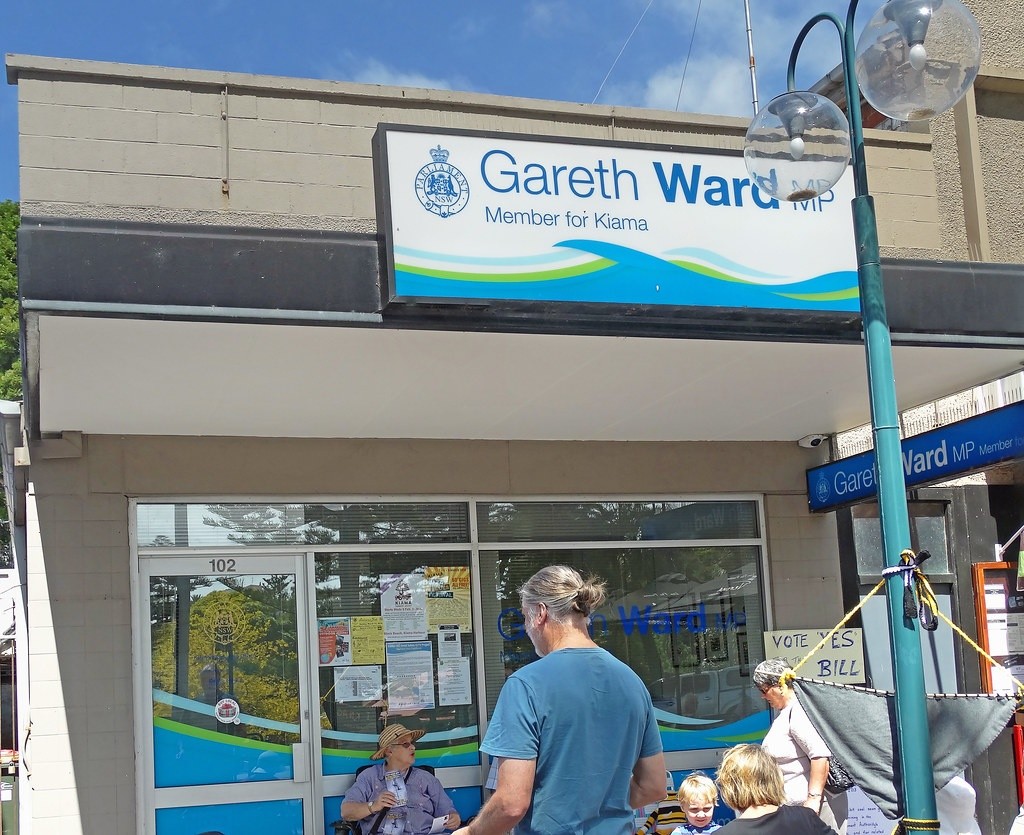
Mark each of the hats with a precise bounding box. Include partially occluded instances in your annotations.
[371,724,426,761]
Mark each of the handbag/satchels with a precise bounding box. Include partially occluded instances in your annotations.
[825,756,855,793]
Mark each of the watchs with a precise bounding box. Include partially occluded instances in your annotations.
[367,801,378,814]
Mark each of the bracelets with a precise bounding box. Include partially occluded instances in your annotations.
[808,793,822,796]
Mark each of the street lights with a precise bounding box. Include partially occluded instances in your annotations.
[742,0,980,835]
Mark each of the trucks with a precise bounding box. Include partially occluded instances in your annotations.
[641,664,764,722]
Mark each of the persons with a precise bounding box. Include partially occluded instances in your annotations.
[340,723,461,835]
[450,565,668,835]
[710,743,839,835]
[181,664,246,738]
[752,656,848,835]
[670,771,723,835]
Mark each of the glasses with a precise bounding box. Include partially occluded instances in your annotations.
[391,741,418,748]
[761,683,778,694]
